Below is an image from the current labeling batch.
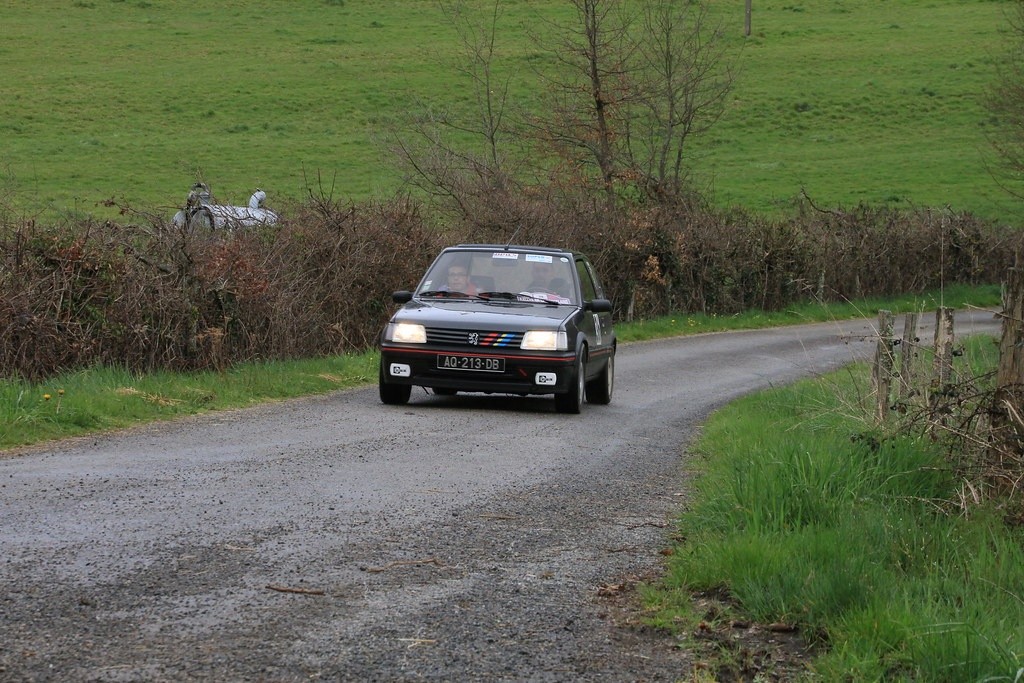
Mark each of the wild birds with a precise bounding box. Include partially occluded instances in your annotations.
[171,182,278,232]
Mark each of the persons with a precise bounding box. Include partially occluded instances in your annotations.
[524,262,570,298]
[433,261,479,300]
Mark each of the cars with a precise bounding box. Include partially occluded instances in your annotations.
[379,244,617,414]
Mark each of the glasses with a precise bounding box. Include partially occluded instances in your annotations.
[448,272,466,276]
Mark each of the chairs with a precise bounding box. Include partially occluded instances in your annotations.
[470,275,496,293]
[548,278,571,299]
